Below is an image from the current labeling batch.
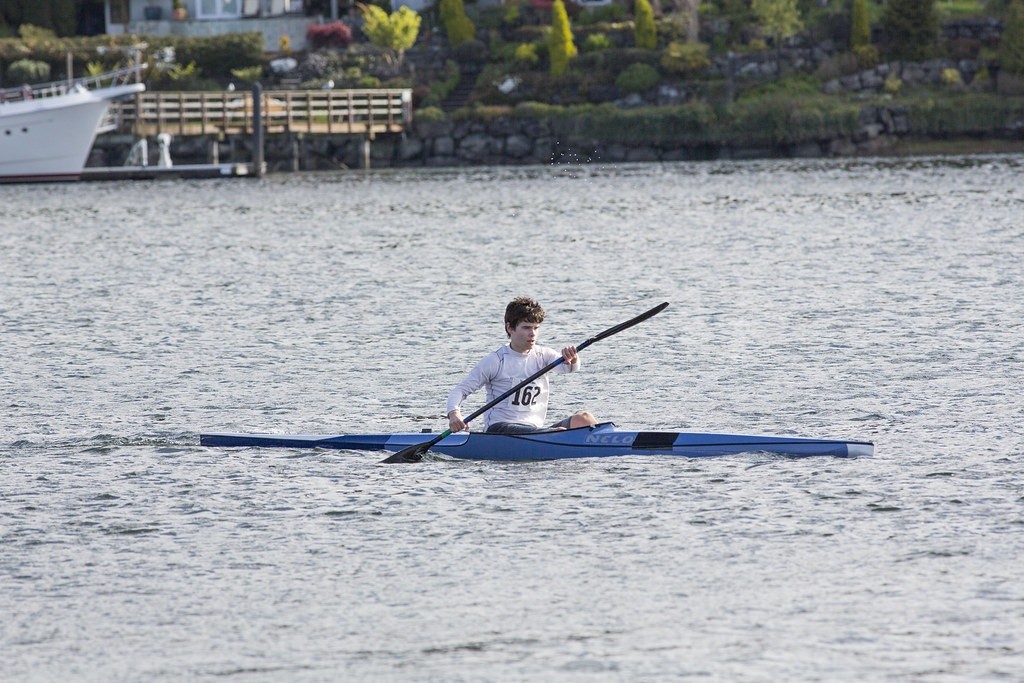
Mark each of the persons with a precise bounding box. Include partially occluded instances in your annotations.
[447,298,598,433]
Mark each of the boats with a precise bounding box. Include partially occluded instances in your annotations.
[0,62,149,185]
[199,422,874,462]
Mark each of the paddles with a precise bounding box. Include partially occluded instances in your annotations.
[379,301,671,464]
